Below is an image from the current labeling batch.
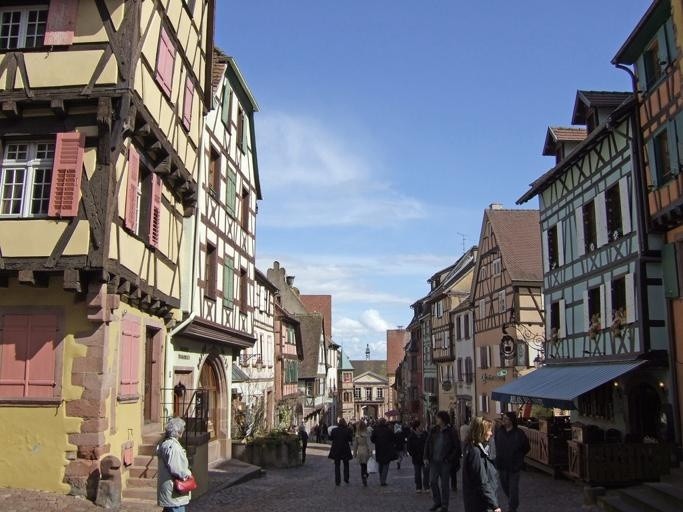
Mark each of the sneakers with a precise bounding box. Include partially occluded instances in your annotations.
[415,488,430,494]
[429,504,449,512]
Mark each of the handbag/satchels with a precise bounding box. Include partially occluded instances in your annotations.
[366,456,379,474]
[174,475,197,495]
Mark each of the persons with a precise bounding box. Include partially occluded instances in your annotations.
[156,416,196,512]
[316,411,531,511]
